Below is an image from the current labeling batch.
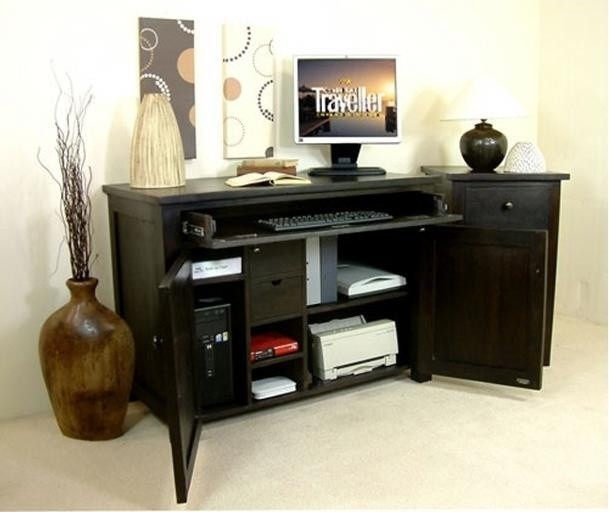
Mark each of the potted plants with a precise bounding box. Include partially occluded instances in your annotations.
[37,70,135,441]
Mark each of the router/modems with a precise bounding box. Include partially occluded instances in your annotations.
[251,375,295,400]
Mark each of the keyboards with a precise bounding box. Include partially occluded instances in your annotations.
[258,207,391,232]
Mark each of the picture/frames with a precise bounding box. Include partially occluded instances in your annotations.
[222,24,275,159]
[139,18,197,159]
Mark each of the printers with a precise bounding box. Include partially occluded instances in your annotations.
[308,317,401,383]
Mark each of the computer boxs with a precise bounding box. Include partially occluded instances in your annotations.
[192,296,235,414]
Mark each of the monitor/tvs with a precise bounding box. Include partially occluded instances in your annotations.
[294,56,401,178]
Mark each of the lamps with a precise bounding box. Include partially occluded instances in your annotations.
[440,61,538,172]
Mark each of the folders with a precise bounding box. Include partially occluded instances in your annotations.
[320,233,337,307]
[305,237,321,307]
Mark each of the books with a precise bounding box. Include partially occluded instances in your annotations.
[236,166,298,177]
[224,170,311,187]
[241,158,299,169]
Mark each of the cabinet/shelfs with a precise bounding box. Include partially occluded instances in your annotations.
[101,176,547,504]
[419,165,569,365]
